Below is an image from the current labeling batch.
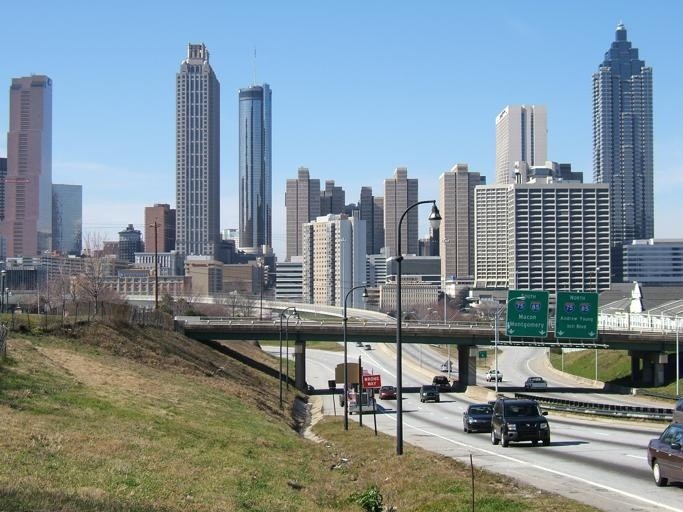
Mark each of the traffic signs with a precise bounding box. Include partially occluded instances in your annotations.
[506,289,548,338]
[555,291,599,340]
[478,351,487,359]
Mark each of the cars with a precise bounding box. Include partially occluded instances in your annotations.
[338,360,552,451]
[643,397,682,488]
[355,342,373,350]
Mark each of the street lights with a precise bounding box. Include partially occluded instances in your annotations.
[150,217,161,310]
[340,285,369,429]
[391,198,442,456]
[277,306,299,410]
